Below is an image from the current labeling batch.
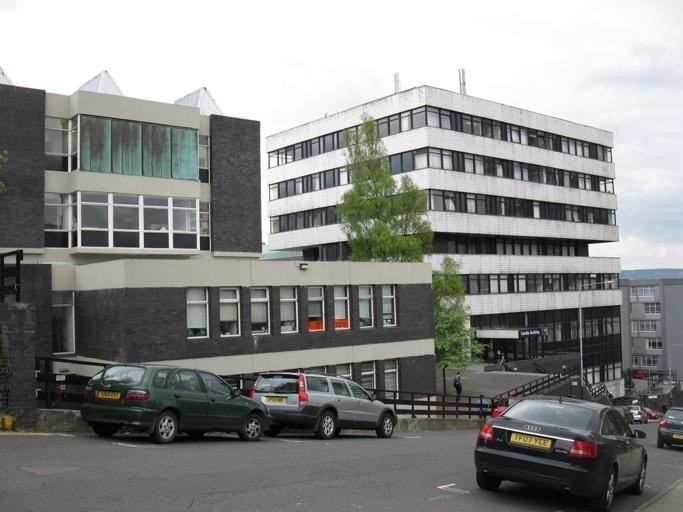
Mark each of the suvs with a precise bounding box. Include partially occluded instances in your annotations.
[251,372,398,440]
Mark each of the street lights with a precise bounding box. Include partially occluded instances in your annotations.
[578,280,613,398]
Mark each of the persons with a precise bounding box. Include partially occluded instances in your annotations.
[477,394,486,419]
[609,392,613,400]
[662,402,667,414]
[497,350,506,371]
[454,372,463,400]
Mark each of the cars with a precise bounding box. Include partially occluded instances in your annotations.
[658,407,683,448]
[79,364,273,443]
[474,394,657,511]
